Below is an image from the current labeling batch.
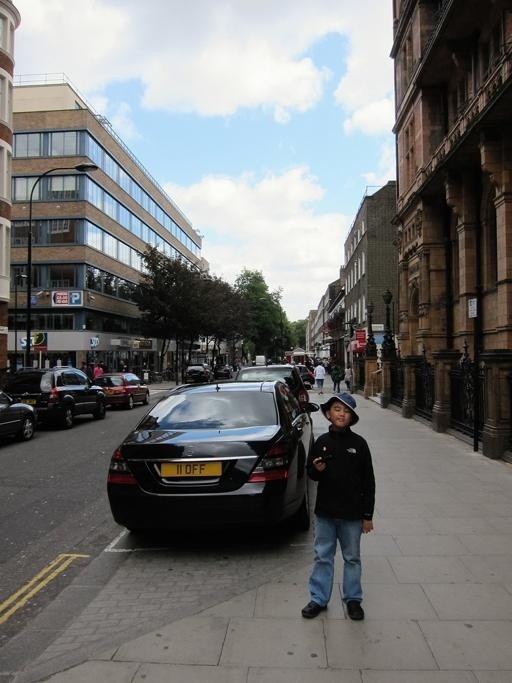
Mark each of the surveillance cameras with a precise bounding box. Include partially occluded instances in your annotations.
[46,291,49,297]
[37,291,43,296]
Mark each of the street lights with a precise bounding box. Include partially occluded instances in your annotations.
[26,161,100,367]
[362,299,379,358]
[12,272,28,372]
[378,287,398,361]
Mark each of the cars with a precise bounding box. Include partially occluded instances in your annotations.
[293,363,316,385]
[251,355,274,365]
[92,371,155,411]
[230,363,312,412]
[106,381,322,552]
[213,363,235,378]
[0,382,42,446]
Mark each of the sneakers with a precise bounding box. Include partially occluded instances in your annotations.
[346,600,365,620]
[301,600,328,618]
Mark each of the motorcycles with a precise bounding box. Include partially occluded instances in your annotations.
[142,362,162,383]
[162,362,176,383]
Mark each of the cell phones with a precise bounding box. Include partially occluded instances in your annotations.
[315,455,333,464]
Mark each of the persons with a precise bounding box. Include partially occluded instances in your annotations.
[302,392,375,620]
[331,362,346,392]
[313,363,328,393]
[83,362,109,382]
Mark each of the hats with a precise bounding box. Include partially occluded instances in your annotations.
[320,392,359,426]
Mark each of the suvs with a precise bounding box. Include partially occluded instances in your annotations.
[181,362,216,384]
[5,363,109,429]
